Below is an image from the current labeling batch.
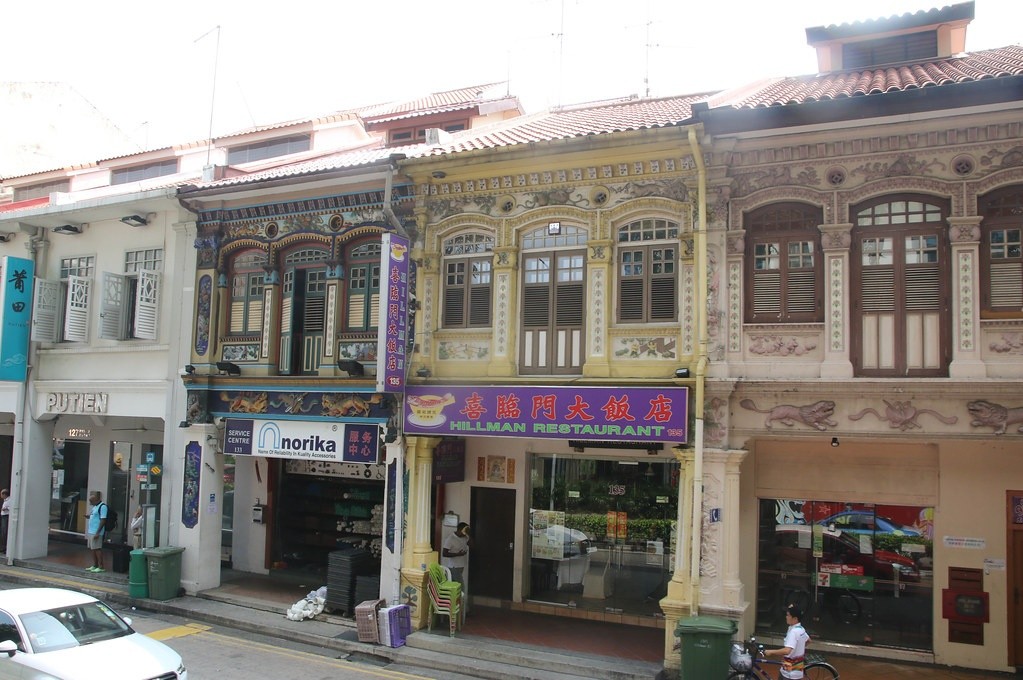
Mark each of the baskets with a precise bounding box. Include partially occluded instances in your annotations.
[729,641,757,672]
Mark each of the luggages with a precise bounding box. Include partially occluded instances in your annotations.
[112,542,133,573]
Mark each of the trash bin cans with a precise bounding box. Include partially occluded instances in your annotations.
[143,545,186,600]
[129,549,149,598]
[673,616,738,680]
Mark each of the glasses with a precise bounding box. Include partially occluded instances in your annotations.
[788,604,796,612]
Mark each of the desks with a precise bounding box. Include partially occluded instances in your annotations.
[556,546,599,589]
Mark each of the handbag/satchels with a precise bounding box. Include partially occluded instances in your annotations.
[99,503,118,532]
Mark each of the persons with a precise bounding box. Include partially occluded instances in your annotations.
[0,489,10,554]
[764,607,812,680]
[84,495,107,573]
[442,522,474,592]
[131,507,144,549]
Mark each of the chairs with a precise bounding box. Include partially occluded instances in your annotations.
[427,562,465,637]
[580,549,617,600]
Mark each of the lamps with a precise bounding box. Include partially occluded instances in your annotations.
[51,223,96,235]
[831,437,840,446]
[417,369,440,377]
[386,428,398,443]
[338,359,366,376]
[0,232,15,242]
[548,222,561,236]
[185,365,198,374]
[216,362,241,376]
[676,368,689,378]
[179,421,191,427]
[118,216,147,227]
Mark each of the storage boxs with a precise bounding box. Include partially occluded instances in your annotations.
[355,598,410,648]
[334,484,374,518]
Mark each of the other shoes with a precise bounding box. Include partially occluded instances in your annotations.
[91,568,106,572]
[0,547,6,552]
[4,552,6,554]
[86,566,95,570]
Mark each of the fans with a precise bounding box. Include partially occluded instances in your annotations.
[112,417,164,433]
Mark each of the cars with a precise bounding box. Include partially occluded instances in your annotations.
[529,507,592,561]
[0,587,188,680]
[775,525,922,596]
[816,510,922,536]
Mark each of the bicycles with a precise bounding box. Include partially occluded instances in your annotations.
[726,635,840,680]
